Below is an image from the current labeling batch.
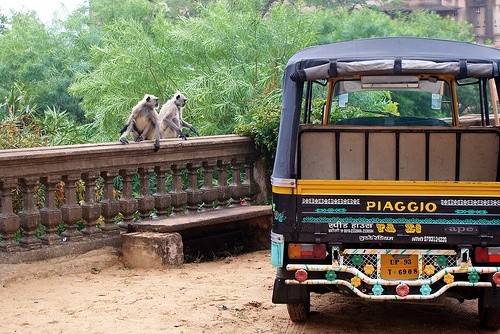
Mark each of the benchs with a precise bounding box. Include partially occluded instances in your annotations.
[120,206,272,269]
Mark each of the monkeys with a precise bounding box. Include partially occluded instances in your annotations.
[159,93,200,138]
[119,94,161,152]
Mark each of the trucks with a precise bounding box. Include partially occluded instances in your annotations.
[270,37,500,326]
